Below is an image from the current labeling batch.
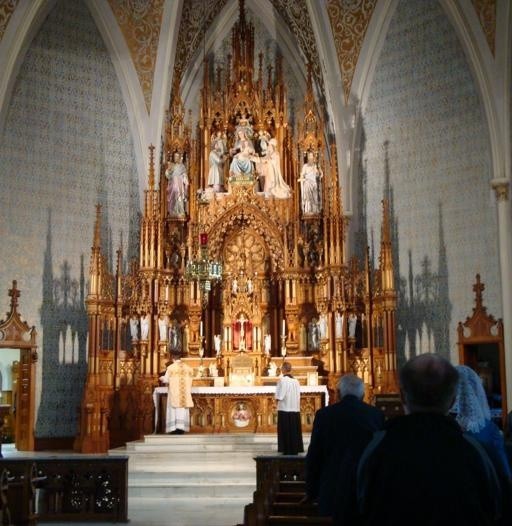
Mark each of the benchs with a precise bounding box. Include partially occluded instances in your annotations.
[236,470,344,525]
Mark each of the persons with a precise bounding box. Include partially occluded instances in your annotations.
[163,150,190,217]
[157,350,195,437]
[297,149,324,215]
[206,117,291,199]
[273,361,305,456]
[302,373,386,526]
[442,364,510,496]
[352,350,509,526]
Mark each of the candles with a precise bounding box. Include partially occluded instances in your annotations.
[200,320,204,337]
[283,319,285,337]
